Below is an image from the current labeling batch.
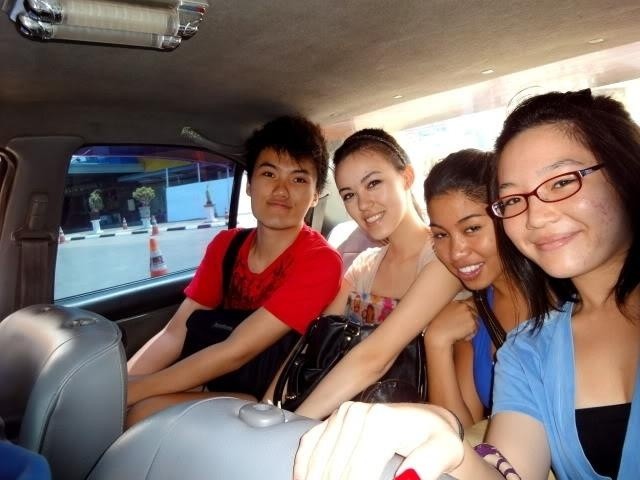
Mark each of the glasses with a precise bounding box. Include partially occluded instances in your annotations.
[486,164,605,219]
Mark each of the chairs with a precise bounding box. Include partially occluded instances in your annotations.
[0,303,128,479]
[325,220,382,276]
[85,396,456,480]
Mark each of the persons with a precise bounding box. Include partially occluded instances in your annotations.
[292,89,639,480]
[424,148,559,429]
[261,128,462,421]
[125,116,343,428]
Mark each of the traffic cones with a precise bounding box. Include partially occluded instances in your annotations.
[59,227,68,243]
[122,217,129,229]
[148,237,169,278]
[150,215,161,236]
[224,207,229,224]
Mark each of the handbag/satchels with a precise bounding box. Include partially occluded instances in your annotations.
[179,308,425,420]
